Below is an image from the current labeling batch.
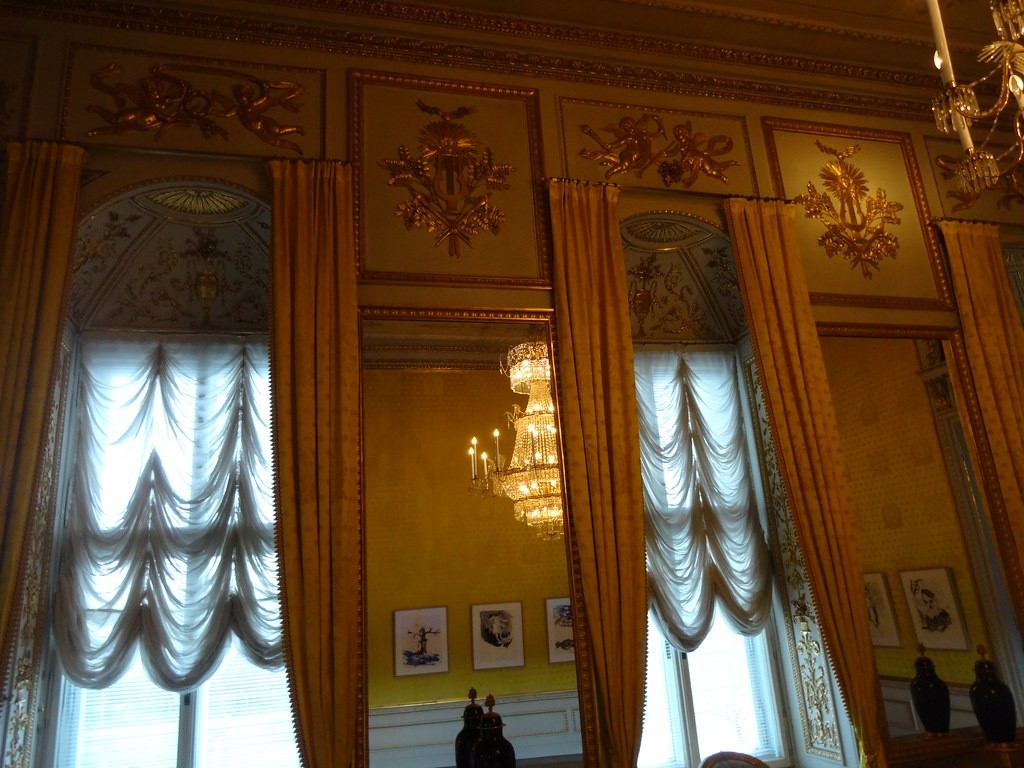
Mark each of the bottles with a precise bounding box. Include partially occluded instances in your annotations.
[908,644,951,734]
[467,694,516,768]
[969,643,1016,743]
[454,686,484,768]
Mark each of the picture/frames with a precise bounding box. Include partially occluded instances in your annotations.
[55,37,327,163]
[0,32,40,143]
[470,601,527,670]
[760,115,955,313]
[925,135,1024,228]
[344,66,555,291]
[558,96,760,200]
[861,571,902,649]
[392,605,449,678]
[544,595,574,664]
[898,565,972,652]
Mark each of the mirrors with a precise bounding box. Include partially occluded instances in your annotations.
[355,304,598,768]
[819,323,1024,756]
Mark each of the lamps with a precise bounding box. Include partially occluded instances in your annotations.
[923,0,1024,215]
[467,323,566,543]
